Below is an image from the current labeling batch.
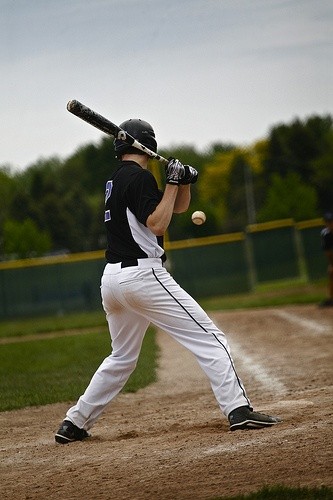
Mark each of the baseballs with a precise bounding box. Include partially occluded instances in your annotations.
[191,211,206,225]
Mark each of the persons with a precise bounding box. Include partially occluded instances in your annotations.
[54,118,282,445]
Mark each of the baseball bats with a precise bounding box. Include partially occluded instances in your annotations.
[68,98,171,167]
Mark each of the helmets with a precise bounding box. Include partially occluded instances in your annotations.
[113,119,157,159]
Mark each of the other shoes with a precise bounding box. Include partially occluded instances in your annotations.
[228,411,281,432]
[55,420,92,443]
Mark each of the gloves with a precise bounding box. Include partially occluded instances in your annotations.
[179,165,199,184]
[164,156,185,186]
